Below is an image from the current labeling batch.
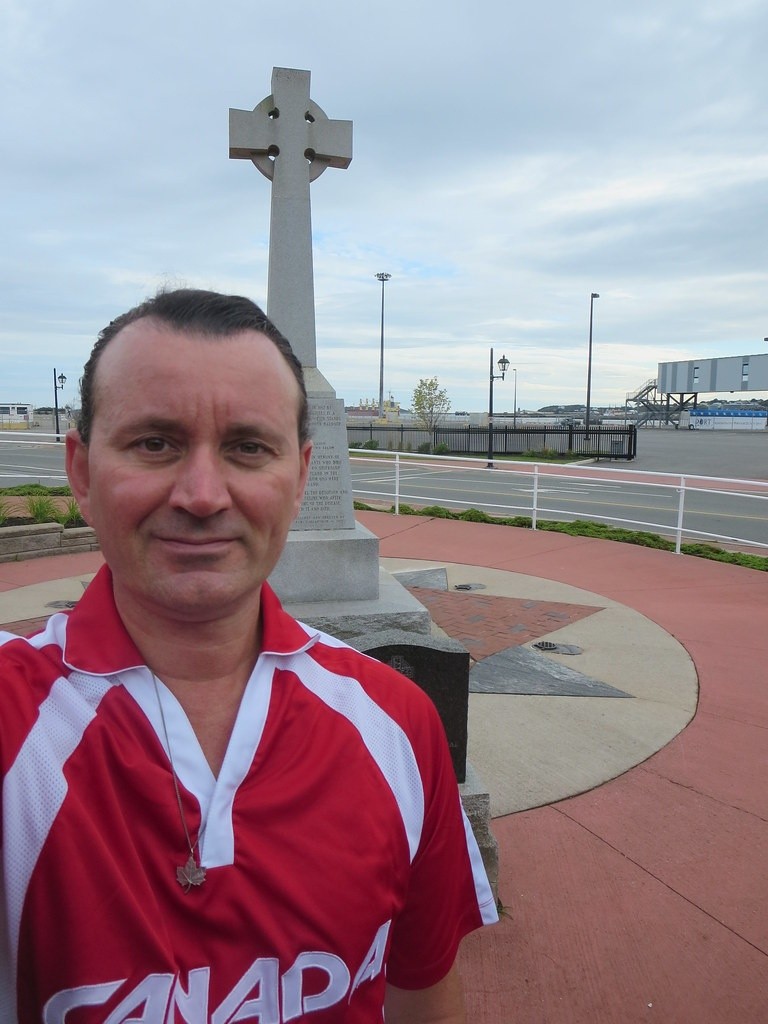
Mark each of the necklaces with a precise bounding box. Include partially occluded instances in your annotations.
[149,668,207,894]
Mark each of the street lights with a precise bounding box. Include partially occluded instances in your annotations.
[513,369,516,429]
[54,368,67,442]
[374,271,391,418]
[485,348,510,469]
[583,293,600,440]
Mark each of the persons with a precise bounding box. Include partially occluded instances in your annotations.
[0,289,500,1024]
